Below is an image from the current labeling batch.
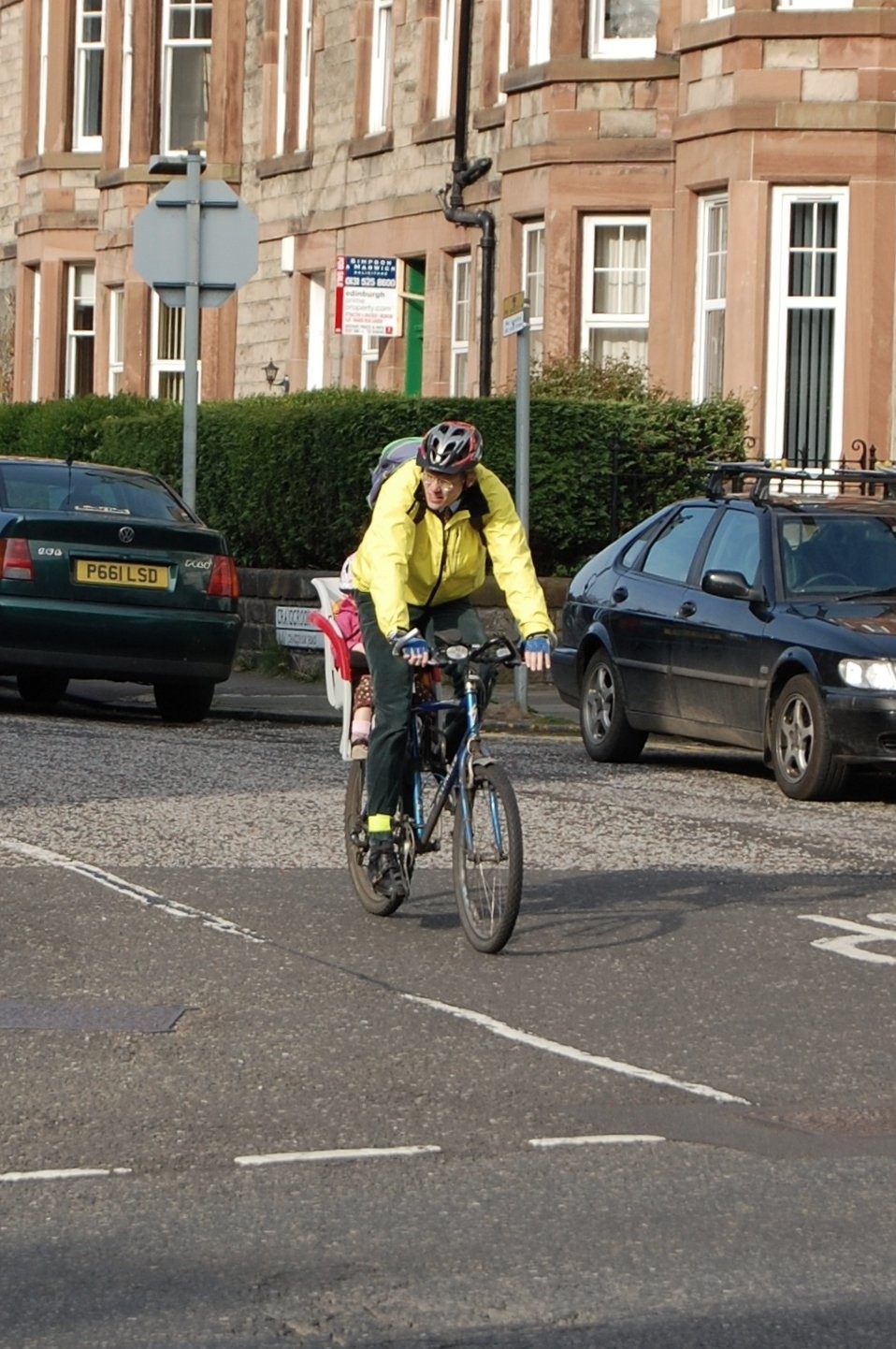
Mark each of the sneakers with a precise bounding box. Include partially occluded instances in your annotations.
[419,739,450,783]
[366,842,407,896]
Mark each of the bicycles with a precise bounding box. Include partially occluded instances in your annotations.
[310,576,553,957]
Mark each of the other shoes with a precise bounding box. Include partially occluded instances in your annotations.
[350,725,369,747]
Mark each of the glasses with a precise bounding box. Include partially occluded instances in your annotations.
[420,466,468,492]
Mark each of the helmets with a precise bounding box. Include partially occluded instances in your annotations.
[338,553,357,592]
[415,421,484,476]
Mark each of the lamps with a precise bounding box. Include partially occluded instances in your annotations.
[261,359,290,393]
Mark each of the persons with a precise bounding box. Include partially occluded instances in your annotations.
[351,422,558,898]
[335,553,438,760]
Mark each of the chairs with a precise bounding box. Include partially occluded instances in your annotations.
[59,493,106,511]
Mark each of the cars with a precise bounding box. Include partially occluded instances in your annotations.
[547,461,895,806]
[0,453,244,725]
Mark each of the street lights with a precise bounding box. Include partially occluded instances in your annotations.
[146,142,209,513]
